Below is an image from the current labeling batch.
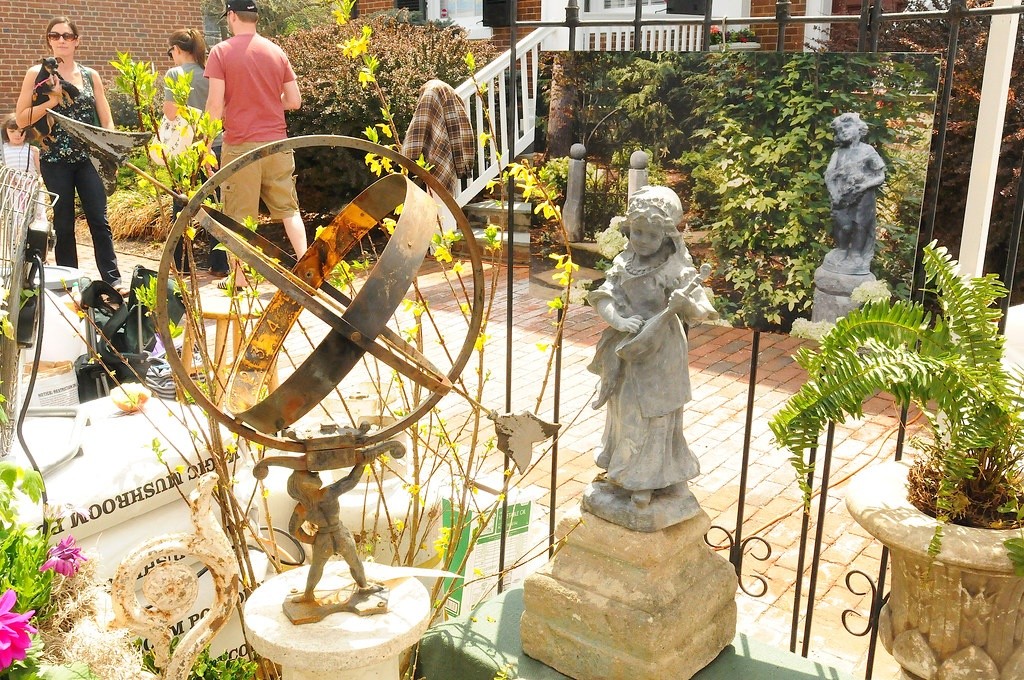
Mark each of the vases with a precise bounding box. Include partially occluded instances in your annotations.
[709,42,761,53]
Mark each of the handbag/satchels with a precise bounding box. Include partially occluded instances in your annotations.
[150,66,202,166]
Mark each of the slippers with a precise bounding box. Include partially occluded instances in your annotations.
[216,277,242,291]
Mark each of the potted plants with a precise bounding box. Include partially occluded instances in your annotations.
[769,241,1024,680]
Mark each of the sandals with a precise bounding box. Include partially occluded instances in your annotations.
[111,284,130,297]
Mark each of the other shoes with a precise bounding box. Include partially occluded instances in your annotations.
[173,343,208,383]
[206,266,228,278]
[168,266,191,275]
[146,358,177,400]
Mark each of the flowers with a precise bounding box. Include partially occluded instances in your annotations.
[710,27,759,43]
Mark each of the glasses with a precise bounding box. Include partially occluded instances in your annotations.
[47,31,78,42]
[168,47,174,60]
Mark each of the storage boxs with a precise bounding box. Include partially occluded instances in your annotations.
[440,496,531,619]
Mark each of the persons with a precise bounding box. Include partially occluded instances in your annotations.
[587,185,719,507]
[162,28,230,277]
[16,17,131,297]
[1,113,48,224]
[203,0,309,290]
[822,113,885,275]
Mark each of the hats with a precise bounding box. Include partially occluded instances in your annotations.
[219,0,258,19]
